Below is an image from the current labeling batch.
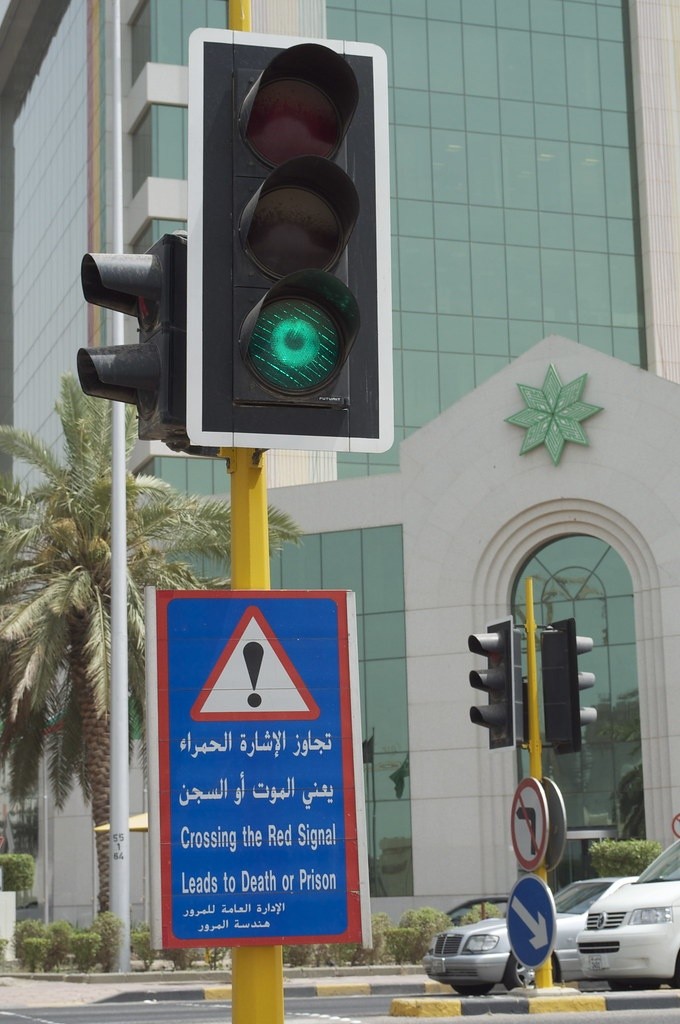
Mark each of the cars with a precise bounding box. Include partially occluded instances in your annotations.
[576,839,680,991]
[423,876,639,996]
[444,895,508,924]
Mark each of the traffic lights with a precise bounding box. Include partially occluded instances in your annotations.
[468,615,524,749]
[186,28,393,454]
[540,619,597,753]
[76,232,221,457]
